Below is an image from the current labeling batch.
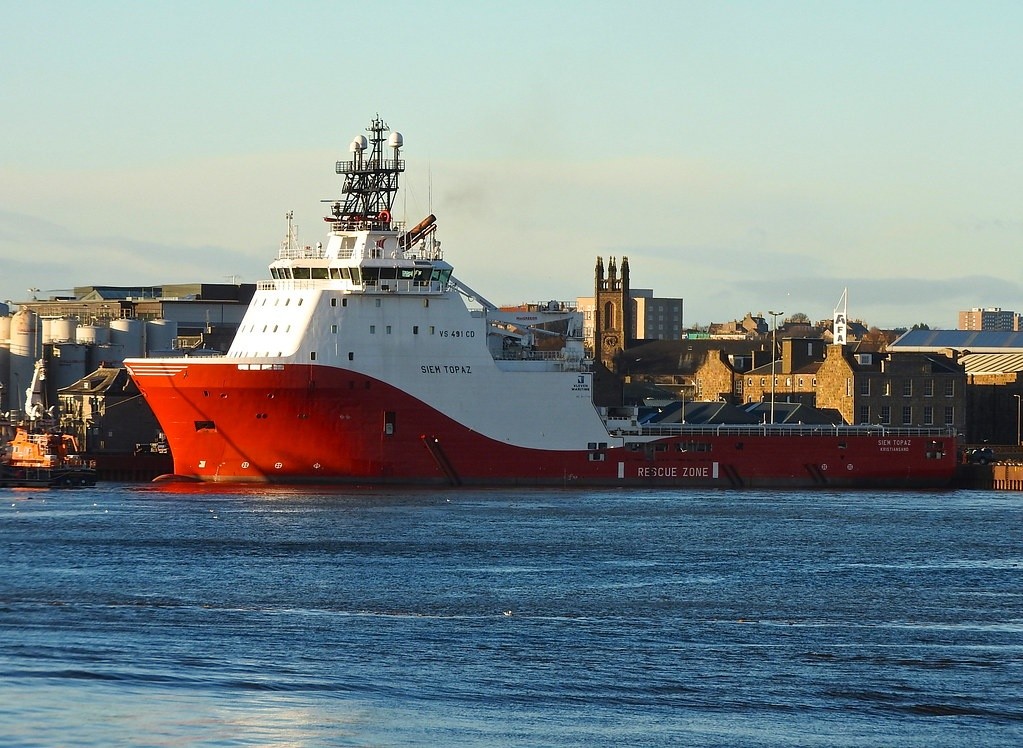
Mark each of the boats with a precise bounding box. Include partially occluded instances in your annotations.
[0,359,96,489]
[121,113,961,489]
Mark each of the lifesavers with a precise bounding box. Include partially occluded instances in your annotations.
[378,211,390,223]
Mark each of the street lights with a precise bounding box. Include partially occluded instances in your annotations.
[1014,395,1020,446]
[768,310,784,423]
